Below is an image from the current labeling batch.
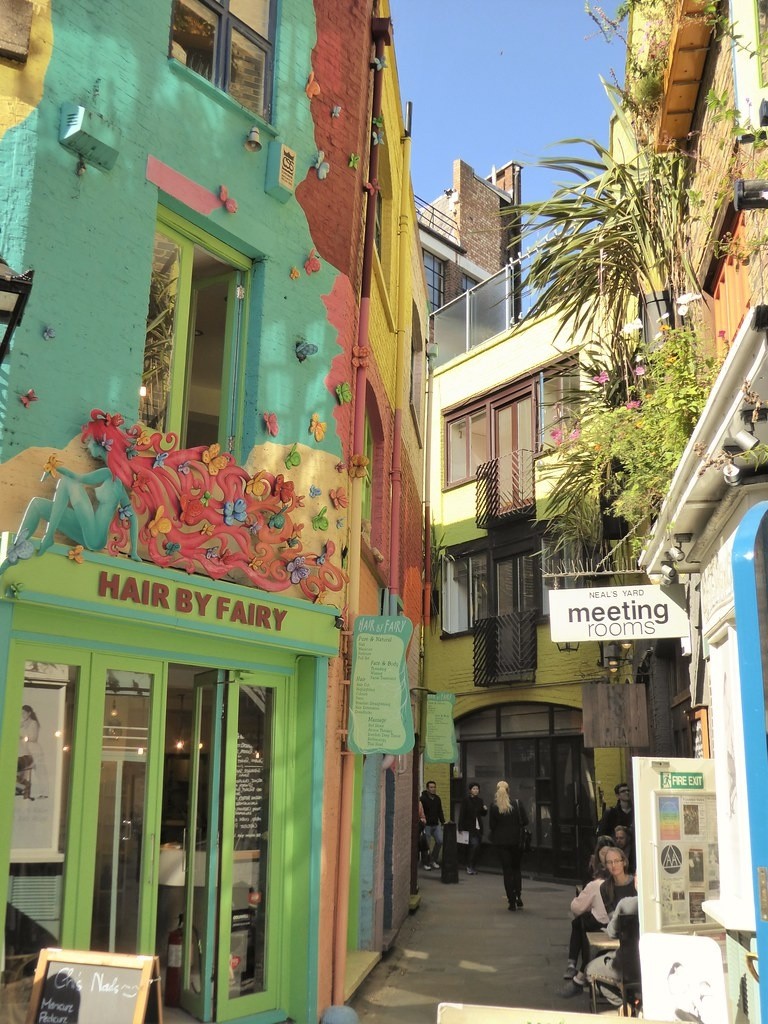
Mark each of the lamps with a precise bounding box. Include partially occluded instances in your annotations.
[244,126,263,154]
[174,694,187,749]
[606,641,619,674]
[110,688,118,717]
[621,638,633,650]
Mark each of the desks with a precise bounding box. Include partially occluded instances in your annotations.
[574,883,595,933]
[586,929,622,1013]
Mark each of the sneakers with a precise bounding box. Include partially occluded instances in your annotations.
[563,959,577,979]
[431,860,440,869]
[466,865,478,875]
[422,864,432,871]
[556,977,585,999]
[617,1001,632,1018]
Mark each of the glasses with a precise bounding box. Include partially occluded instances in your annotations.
[605,858,623,865]
[619,789,631,793]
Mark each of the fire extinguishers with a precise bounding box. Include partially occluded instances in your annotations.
[163,913,194,1006]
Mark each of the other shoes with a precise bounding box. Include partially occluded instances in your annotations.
[516,897,523,906]
[508,901,517,911]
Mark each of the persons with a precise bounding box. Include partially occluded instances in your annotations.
[555,780,651,1019]
[1,408,349,604]
[421,780,446,872]
[15,705,39,796]
[457,783,487,876]
[490,780,529,911]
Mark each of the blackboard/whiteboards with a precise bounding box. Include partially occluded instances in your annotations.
[233,685,265,860]
[28,948,162,1024]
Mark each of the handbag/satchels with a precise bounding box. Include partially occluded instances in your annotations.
[517,826,533,852]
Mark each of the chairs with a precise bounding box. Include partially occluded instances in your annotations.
[589,912,643,1017]
